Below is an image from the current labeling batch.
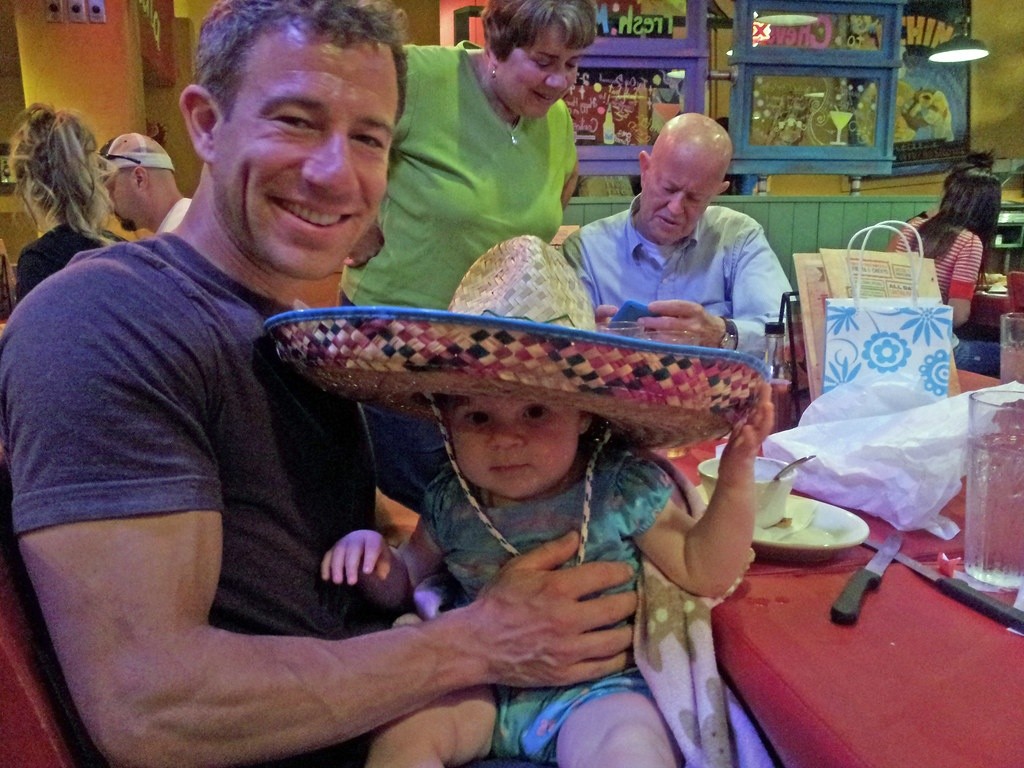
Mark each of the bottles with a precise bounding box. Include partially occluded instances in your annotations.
[758,320,793,434]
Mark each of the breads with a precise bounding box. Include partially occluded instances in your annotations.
[978,272,1008,285]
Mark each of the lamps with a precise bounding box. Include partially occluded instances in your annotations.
[927,15,989,62]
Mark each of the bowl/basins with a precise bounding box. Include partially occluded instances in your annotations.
[697,455,797,528]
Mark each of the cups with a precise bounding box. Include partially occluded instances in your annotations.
[1000,312,1024,386]
[594,320,699,458]
[964,390,1024,588]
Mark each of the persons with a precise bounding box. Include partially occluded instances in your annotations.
[338,0,598,310]
[101,134,192,237]
[264,235,773,768]
[561,113,792,360]
[12,103,129,304]
[0,0,638,768]
[886,152,1000,376]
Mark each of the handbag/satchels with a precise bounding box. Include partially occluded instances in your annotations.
[821,219,954,399]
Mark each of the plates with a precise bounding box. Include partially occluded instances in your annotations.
[976,283,1007,287]
[984,288,1008,294]
[693,485,871,562]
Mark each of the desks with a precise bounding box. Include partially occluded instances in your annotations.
[665,275,1024,768]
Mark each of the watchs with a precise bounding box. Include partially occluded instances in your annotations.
[720,316,736,350]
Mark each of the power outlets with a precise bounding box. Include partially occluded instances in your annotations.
[46,0,63,23]
[67,0,87,24]
[88,0,106,24]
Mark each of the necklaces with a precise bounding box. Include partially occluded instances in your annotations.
[510,118,520,144]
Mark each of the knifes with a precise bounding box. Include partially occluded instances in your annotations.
[829,528,904,624]
[861,539,1024,634]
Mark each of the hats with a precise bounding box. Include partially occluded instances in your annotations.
[98,133,175,172]
[262,235,770,453]
